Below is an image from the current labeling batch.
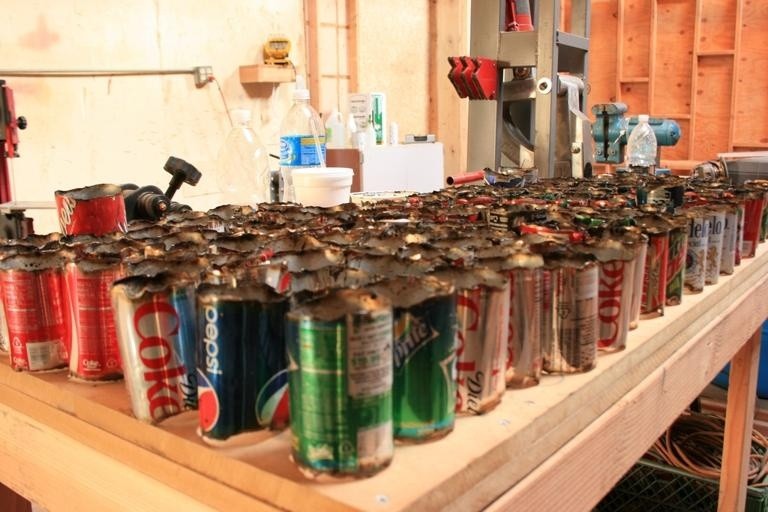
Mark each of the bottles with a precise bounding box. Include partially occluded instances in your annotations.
[221,108,271,203]
[278,86,326,204]
[327,94,399,150]
[625,113,658,175]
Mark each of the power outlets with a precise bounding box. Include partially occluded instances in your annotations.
[192,64,214,85]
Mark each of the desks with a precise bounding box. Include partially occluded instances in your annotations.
[3,236,767,512]
[316,140,445,198]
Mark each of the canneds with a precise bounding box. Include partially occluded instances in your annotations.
[348,91,386,146]
[1,165,767,483]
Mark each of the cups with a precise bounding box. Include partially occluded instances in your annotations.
[349,189,418,206]
